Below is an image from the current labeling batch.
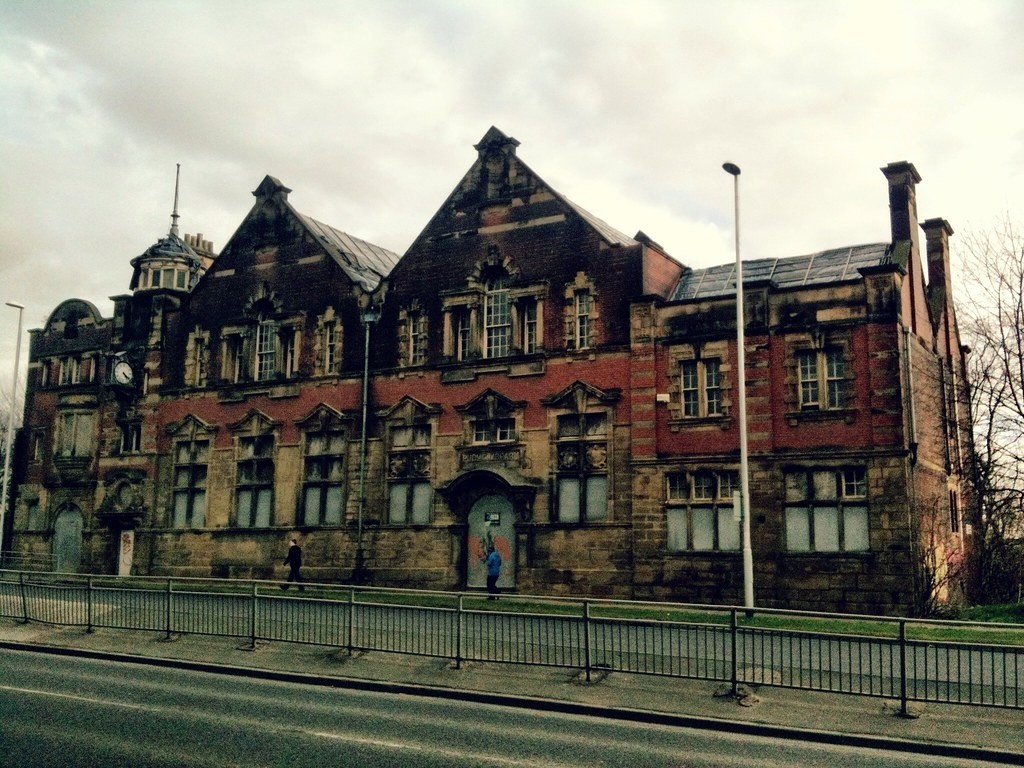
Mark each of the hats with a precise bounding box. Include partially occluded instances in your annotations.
[292,539,297,544]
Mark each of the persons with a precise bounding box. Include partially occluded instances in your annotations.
[487,547,502,600]
[280,539,305,592]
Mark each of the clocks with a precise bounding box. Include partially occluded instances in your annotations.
[115,361,133,384]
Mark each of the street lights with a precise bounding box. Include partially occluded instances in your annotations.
[721,160,756,612]
[1,301,25,550]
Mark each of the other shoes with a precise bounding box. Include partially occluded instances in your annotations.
[497,590,501,600]
[280,584,286,591]
[487,597,495,600]
